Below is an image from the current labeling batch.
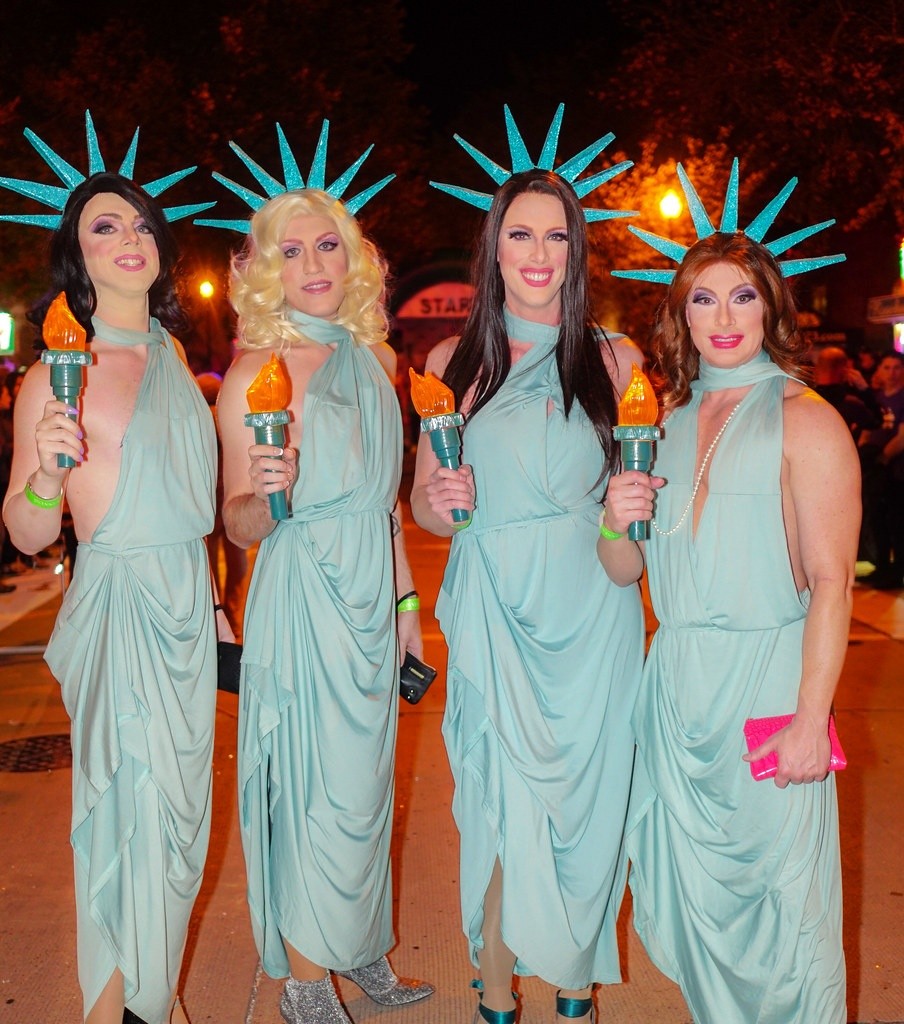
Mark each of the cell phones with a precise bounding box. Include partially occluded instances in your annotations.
[399,650,438,704]
[217,641,242,694]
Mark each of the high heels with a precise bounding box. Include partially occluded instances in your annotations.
[278,970,353,1024]
[327,948,434,1005]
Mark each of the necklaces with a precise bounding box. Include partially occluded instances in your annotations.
[645,401,740,535]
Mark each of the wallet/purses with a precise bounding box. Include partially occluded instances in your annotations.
[397,652,437,705]
[215,640,250,693]
[744,712,846,780]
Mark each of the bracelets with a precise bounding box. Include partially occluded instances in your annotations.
[397,598,420,614]
[395,588,420,605]
[24,472,64,507]
[449,511,474,530]
[212,603,227,612]
[598,507,625,542]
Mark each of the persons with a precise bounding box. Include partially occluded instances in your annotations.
[410,103,644,1024]
[0,312,904,593]
[596,158,862,1023]
[2,110,237,1024]
[188,118,435,1024]
